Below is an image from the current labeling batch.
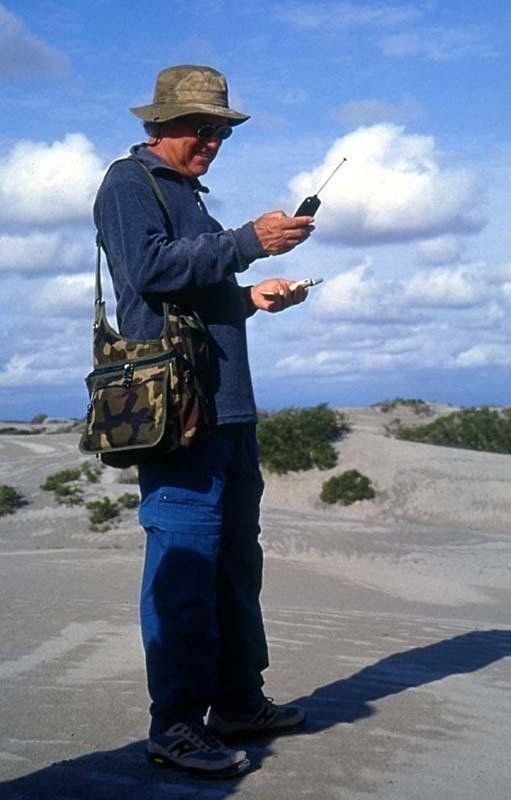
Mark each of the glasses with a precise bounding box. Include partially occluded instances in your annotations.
[188,119,233,141]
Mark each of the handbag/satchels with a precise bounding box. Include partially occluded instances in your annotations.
[78,293,227,468]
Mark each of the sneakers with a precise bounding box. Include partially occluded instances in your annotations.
[146,714,251,780]
[206,686,308,738]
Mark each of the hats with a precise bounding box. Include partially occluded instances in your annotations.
[129,65,251,127]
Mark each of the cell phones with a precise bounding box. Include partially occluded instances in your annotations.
[293,194,321,218]
[261,277,323,302]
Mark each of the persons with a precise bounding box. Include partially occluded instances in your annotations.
[92,64,316,783]
